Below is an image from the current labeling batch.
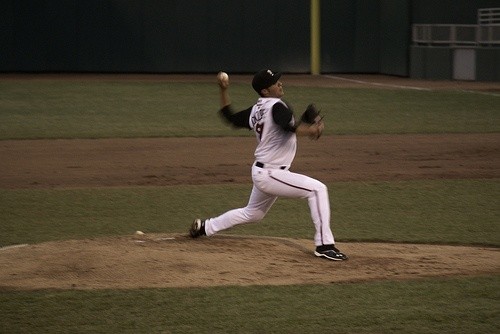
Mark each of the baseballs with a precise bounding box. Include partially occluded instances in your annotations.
[215,72,230,86]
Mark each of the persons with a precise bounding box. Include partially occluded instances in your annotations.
[190,68,349,262]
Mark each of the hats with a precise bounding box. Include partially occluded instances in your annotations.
[252,69,281,91]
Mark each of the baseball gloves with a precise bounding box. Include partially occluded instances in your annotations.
[300,103,325,142]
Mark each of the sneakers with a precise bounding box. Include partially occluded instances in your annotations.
[314,244,346,261]
[190,218,205,238]
